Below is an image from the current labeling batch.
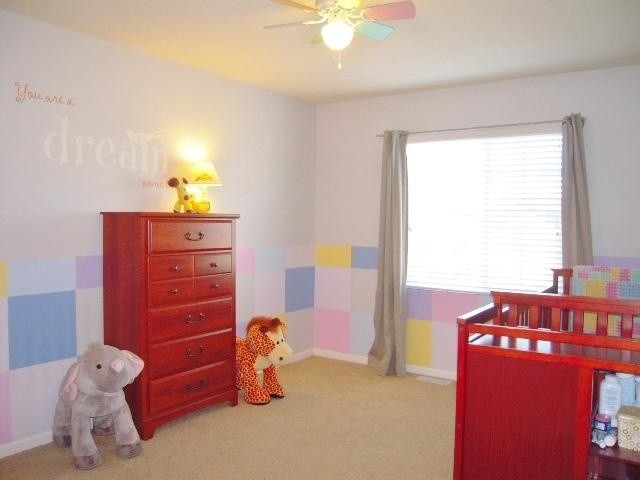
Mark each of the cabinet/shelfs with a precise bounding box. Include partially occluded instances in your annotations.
[100,212,240,441]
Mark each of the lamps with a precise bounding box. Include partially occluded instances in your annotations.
[184,161,223,214]
[322,16,353,71]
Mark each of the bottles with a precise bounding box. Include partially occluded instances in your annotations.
[598,373,621,429]
[594,413,611,432]
[616,372,637,407]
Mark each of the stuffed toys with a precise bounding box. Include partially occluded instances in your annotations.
[52,338,146,472]
[235,312,295,406]
[166,174,200,213]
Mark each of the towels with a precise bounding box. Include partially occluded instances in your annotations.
[568,265,640,339]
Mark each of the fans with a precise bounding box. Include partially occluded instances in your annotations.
[263,0,415,44]
